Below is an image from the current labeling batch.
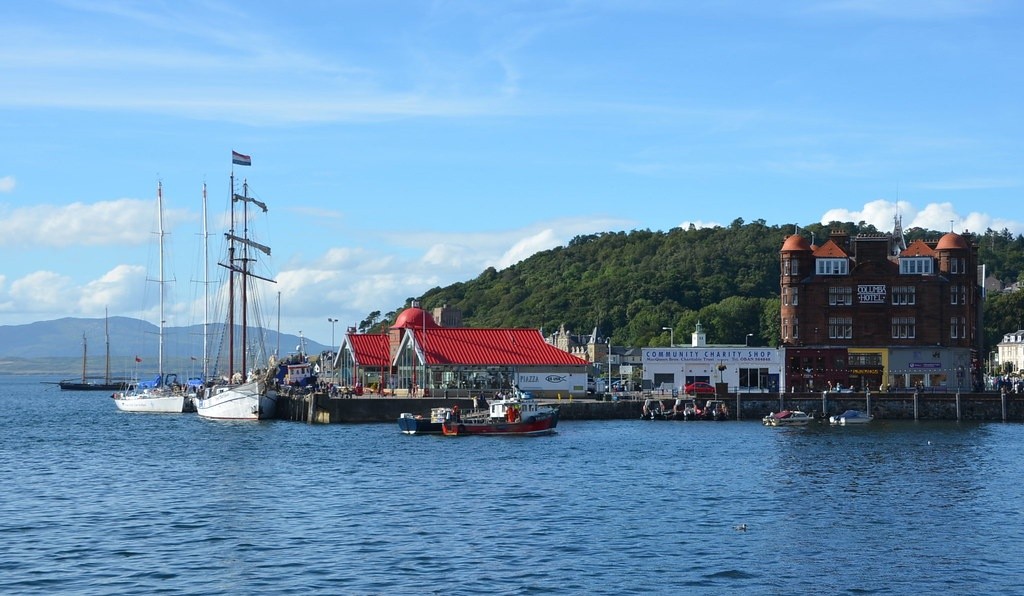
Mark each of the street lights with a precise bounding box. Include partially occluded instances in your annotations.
[605,336,612,394]
[663,327,673,347]
[746,334,753,346]
[989,351,995,380]
[328,318,338,385]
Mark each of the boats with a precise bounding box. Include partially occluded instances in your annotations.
[397,407,491,435]
[137,374,160,388]
[640,394,732,421]
[762,404,814,427]
[830,410,874,427]
[441,380,559,437]
[186,338,204,389]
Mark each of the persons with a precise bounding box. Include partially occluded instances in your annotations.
[406,381,412,398]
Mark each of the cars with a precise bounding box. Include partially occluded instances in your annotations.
[605,379,639,391]
[684,382,714,395]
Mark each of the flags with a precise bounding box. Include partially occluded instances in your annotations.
[233,151,251,166]
[135,355,142,363]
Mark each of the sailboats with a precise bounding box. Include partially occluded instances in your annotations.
[59,308,135,392]
[110,180,188,415]
[184,149,278,421]
